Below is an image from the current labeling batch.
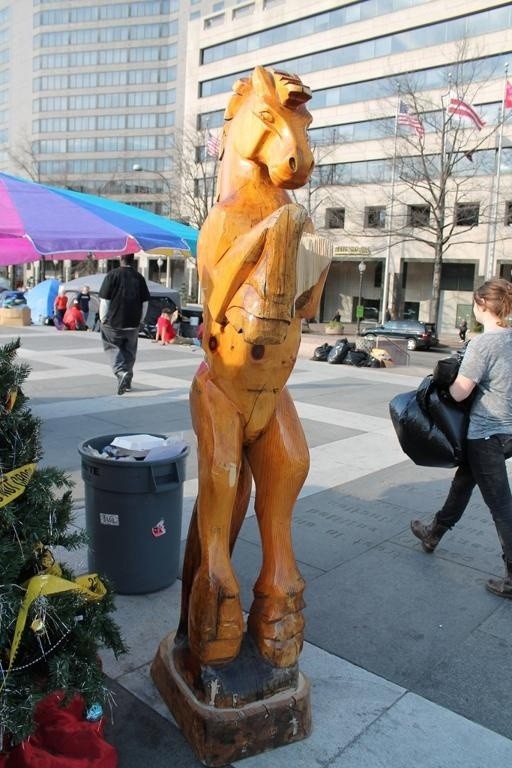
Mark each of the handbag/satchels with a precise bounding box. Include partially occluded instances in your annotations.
[461,327,467,333]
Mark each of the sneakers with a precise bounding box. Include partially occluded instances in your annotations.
[125,385,132,392]
[115,370,133,396]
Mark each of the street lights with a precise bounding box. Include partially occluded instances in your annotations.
[131,163,176,217]
[356,256,368,335]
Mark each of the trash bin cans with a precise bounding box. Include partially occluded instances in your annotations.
[182,309,203,338]
[77,432,191,595]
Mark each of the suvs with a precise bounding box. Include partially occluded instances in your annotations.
[360,319,439,350]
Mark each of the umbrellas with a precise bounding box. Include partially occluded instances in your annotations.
[0,171,201,269]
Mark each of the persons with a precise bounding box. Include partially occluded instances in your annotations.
[55,286,90,331]
[151,307,202,346]
[98,252,150,395]
[332,311,341,322]
[410,278,511,598]
[459,319,467,343]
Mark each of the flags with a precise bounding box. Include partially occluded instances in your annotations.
[503,79,512,109]
[396,99,424,139]
[448,98,487,132]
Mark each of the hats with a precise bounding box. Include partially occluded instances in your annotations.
[161,307,173,313]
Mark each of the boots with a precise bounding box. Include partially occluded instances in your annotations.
[410,514,451,554]
[485,554,512,599]
